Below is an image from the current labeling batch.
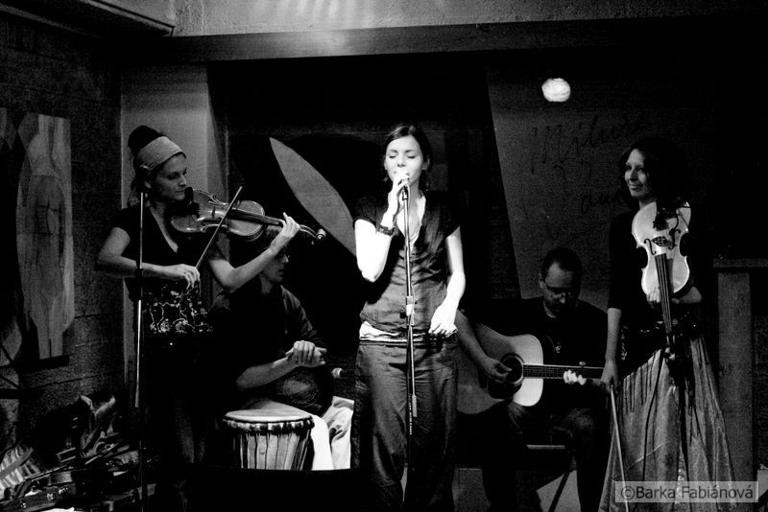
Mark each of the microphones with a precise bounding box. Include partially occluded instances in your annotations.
[397,171,411,199]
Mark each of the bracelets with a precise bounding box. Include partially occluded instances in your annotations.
[376,224,398,239]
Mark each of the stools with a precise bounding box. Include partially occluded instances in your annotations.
[526,442,572,512]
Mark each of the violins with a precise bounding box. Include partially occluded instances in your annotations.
[631,199,696,362]
[171,188,327,244]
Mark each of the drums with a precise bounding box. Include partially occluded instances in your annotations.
[221,408,314,471]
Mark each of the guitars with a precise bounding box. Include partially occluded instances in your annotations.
[457,323,603,414]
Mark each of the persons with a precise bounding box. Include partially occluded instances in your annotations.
[449,246,618,510]
[349,123,466,509]
[598,137,736,511]
[202,224,354,473]
[99,124,297,510]
